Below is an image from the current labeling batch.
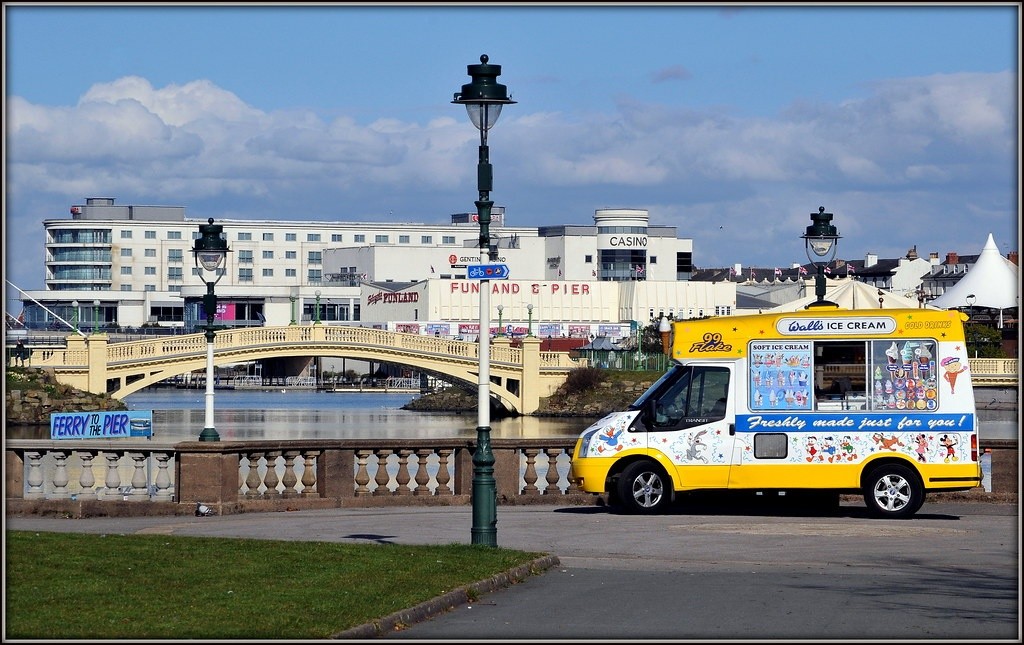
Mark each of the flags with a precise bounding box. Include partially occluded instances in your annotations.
[731,268,737,275]
[824,267,831,273]
[800,266,808,275]
[848,265,854,272]
[361,273,366,279]
[752,271,756,277]
[637,266,643,274]
[775,268,782,275]
[592,270,596,277]
[559,269,562,276]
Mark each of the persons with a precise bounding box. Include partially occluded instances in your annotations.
[14,340,24,367]
[435,329,439,337]
[459,331,464,341]
[562,332,571,338]
[548,335,552,347]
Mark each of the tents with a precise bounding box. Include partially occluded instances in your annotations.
[929,232,1018,328]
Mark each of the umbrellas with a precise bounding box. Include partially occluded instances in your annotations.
[771,280,937,313]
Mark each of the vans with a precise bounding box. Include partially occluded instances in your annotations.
[571,307,994,519]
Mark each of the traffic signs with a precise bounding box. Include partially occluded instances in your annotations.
[466,264,509,280]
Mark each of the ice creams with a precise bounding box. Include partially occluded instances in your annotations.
[659,316,671,355]
[752,371,809,406]
[885,341,932,383]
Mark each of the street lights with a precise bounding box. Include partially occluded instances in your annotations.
[72,301,79,336]
[798,206,843,309]
[497,304,504,337]
[314,289,322,324]
[186,217,236,442]
[93,299,101,334]
[289,291,298,326]
[637,321,644,370]
[526,304,534,337]
[450,53,518,548]
